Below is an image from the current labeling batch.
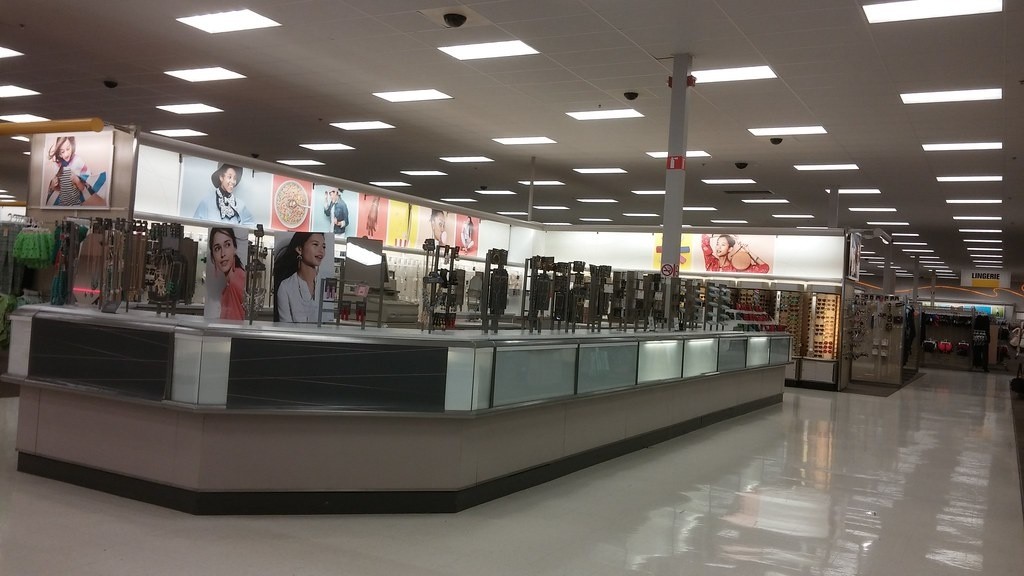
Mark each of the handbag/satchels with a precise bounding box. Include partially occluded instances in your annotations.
[996,321,1024,395]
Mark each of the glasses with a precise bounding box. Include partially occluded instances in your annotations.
[721,287,835,360]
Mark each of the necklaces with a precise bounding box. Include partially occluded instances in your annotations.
[296,271,317,302]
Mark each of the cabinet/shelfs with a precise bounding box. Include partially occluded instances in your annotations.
[737,281,921,392]
[921,305,974,371]
[975,312,1024,378]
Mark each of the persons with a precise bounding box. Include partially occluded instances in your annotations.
[193,162,256,228]
[701,233,770,274]
[323,186,349,238]
[209,228,246,320]
[429,209,447,250]
[46,137,106,206]
[903,305,917,366]
[460,216,475,255]
[273,231,326,323]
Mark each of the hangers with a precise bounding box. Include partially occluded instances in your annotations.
[924,337,971,346]
[17,289,44,306]
[2,212,51,233]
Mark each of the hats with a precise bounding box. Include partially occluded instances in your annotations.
[211,161,243,188]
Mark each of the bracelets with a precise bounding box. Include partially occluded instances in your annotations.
[755,256,759,262]
[366,196,380,237]
[372,203,377,207]
